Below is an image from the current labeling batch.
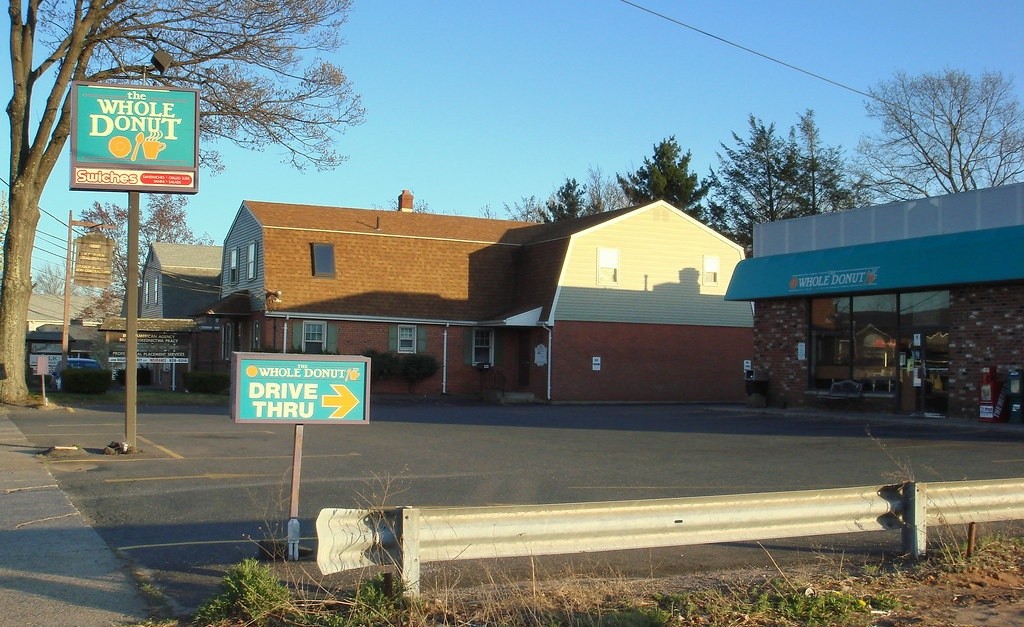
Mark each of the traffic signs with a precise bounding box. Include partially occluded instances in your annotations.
[230,350,371,424]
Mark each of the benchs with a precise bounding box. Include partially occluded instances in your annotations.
[817,378,865,411]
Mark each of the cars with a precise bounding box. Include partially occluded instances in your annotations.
[50,358,101,392]
